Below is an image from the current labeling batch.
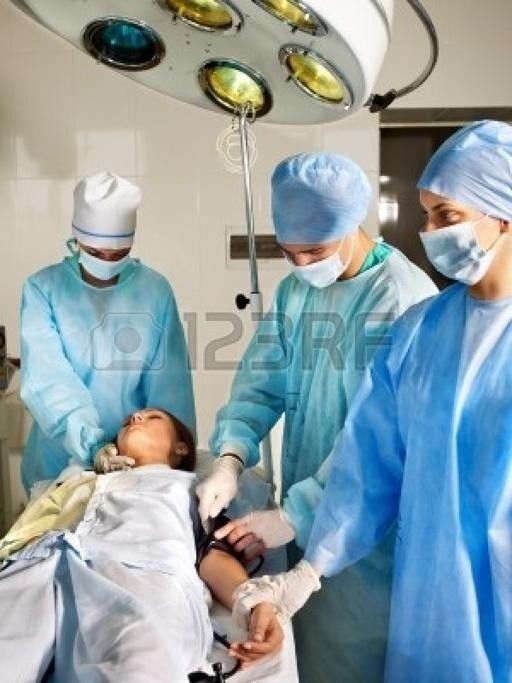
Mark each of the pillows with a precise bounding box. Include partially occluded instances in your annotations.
[190,454,274,522]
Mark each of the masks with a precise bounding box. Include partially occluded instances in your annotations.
[417,213,506,287]
[281,234,356,288]
[78,248,131,281]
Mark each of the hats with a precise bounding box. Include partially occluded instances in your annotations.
[71,171,142,250]
[270,151,372,245]
[415,119,512,224]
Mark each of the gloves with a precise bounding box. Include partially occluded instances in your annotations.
[195,455,245,535]
[231,559,322,632]
[94,444,135,473]
[213,507,296,564]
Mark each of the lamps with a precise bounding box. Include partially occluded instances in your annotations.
[14,0,396,473]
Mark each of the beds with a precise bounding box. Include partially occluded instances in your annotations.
[30,449,298,683]
